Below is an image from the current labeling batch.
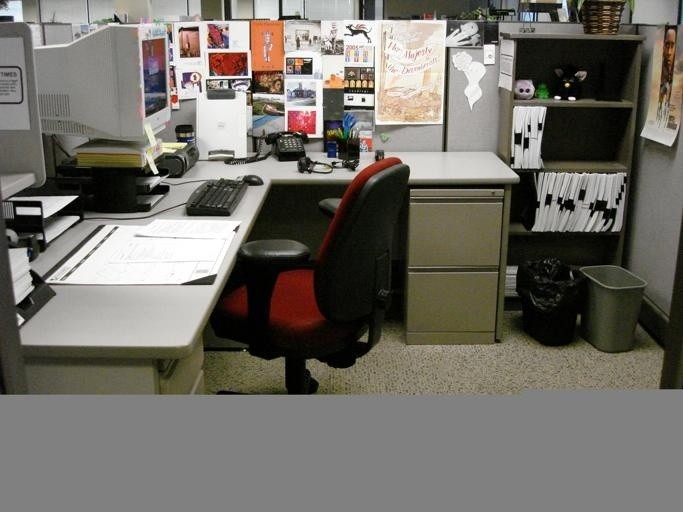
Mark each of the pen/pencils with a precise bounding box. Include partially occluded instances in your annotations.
[324,115,361,159]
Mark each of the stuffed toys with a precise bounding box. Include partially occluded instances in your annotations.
[535,84,549,98]
[555,67,586,101]
[515,81,533,99]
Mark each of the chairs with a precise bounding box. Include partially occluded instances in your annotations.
[208,157,411,395]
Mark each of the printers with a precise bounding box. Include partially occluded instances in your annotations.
[196,89,246,161]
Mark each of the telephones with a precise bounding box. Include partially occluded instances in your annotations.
[265,129,309,160]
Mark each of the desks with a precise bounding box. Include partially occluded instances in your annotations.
[181,140,512,346]
[0,179,271,395]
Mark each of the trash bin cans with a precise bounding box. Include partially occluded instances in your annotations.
[516,256,589,346]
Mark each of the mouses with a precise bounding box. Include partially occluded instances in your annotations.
[242,175,264,186]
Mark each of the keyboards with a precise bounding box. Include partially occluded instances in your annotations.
[186,178,249,217]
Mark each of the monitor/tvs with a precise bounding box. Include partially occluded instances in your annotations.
[32,24,172,153]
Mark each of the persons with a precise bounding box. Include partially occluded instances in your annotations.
[658,30,677,129]
[223,26,228,49]
[296,37,301,50]
[271,78,281,94]
[219,28,223,34]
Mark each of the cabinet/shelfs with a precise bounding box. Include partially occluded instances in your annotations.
[500,22,648,300]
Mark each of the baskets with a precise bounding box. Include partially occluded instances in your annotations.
[582,0,625,35]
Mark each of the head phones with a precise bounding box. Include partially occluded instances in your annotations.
[342,159,359,171]
[297,156,333,175]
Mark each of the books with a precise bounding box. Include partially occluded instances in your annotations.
[524,172,627,232]
[7,248,35,305]
[511,106,547,168]
[506,264,518,295]
